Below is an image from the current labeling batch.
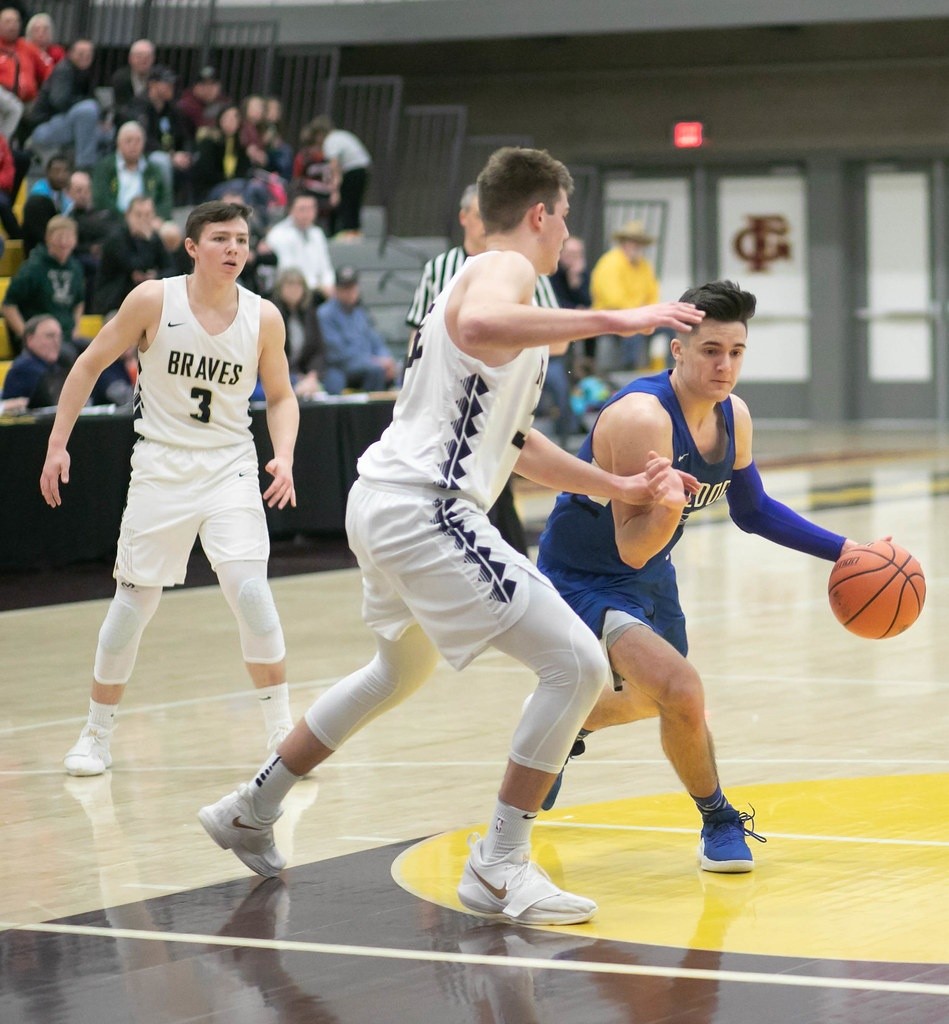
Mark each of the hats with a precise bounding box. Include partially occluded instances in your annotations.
[148,68,176,82]
[613,221,655,244]
[198,66,220,83]
[334,263,361,288]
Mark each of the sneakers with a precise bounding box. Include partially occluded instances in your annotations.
[63,723,119,776]
[264,721,295,754]
[542,739,585,811]
[198,784,288,878]
[457,832,597,925]
[457,926,597,1004]
[208,879,292,984]
[696,805,753,873]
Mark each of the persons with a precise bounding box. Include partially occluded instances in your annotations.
[405,183,568,559]
[195,149,707,926]
[543,220,667,437]
[40,202,299,778]
[532,282,895,872]
[0,6,401,416]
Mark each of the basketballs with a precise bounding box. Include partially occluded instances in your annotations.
[827,539,927,639]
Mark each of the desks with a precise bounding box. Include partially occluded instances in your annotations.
[0,387,531,613]
